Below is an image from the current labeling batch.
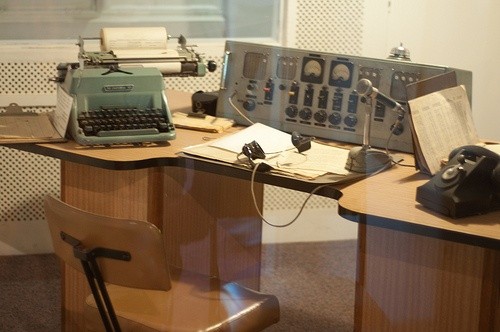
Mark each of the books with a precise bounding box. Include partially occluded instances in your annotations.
[171,112,234,133]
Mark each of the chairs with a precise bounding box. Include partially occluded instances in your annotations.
[44,196,280,332]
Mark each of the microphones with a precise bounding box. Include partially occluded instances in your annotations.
[357,78,406,115]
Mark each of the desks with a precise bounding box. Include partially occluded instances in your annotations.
[0,113,500,332]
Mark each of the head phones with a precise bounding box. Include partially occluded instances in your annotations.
[242,130,311,161]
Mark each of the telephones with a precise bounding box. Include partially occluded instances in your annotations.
[417,145,500,219]
